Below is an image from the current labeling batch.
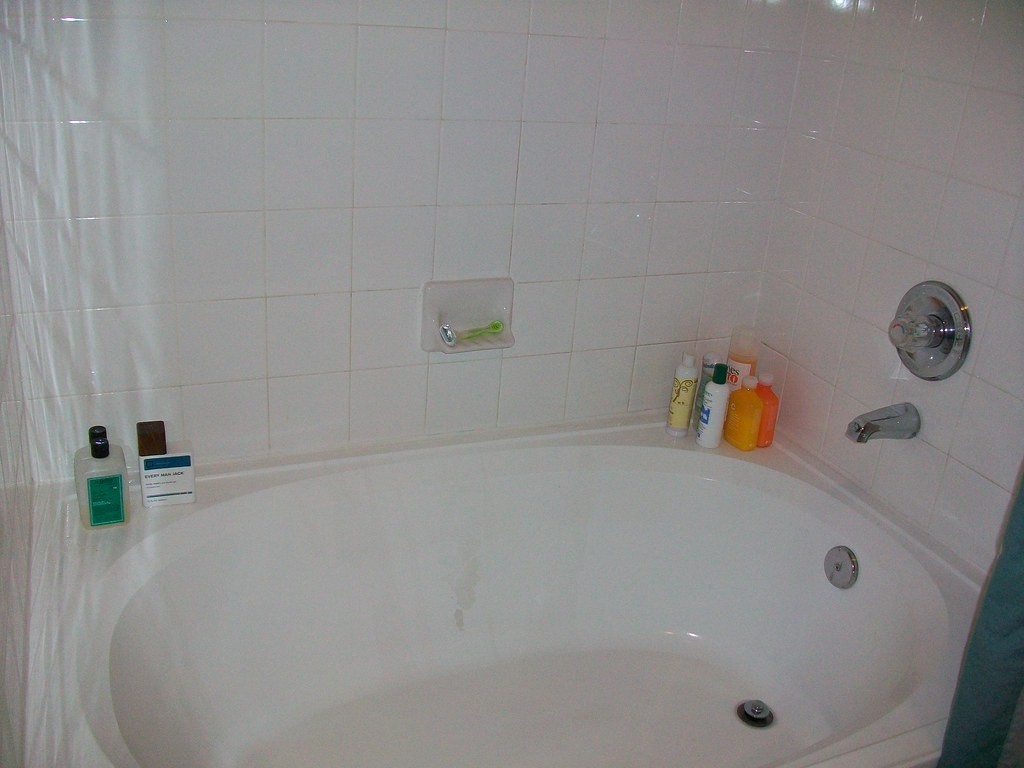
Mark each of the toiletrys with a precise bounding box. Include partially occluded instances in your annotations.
[73,424,132,530]
[665,330,780,451]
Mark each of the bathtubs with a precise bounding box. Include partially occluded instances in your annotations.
[51,436,954,768]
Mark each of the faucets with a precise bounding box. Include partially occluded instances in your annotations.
[844,402,920,445]
